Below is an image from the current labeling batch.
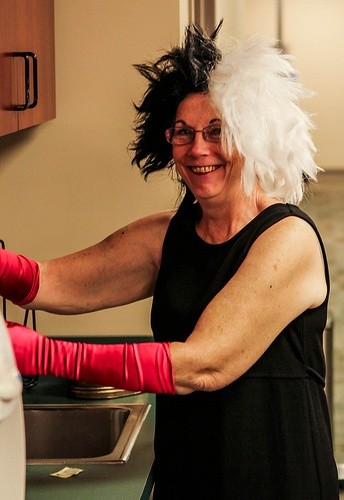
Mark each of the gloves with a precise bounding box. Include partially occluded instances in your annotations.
[3,319,178,396]
[0,248,40,307]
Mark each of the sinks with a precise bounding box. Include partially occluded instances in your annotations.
[23,406,131,459]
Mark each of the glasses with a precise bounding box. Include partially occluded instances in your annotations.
[164,123,224,145]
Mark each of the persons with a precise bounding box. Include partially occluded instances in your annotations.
[1,16,341,500]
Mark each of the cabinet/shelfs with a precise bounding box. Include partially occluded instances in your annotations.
[0,0,55,137]
